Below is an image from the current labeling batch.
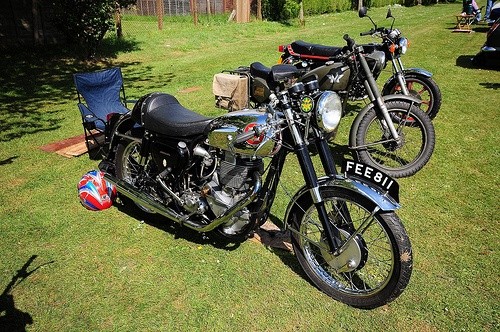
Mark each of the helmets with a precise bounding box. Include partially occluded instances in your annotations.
[77,170,117,211]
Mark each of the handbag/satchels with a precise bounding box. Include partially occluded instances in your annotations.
[212,73,249,111]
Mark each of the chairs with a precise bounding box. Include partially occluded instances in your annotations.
[73,66,142,162]
[454,6,484,30]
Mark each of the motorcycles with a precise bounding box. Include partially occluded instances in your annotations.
[471,0,500,70]
[98,63,414,311]
[277,8,442,128]
[211,35,437,179]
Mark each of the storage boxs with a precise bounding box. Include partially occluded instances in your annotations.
[213,73,249,111]
